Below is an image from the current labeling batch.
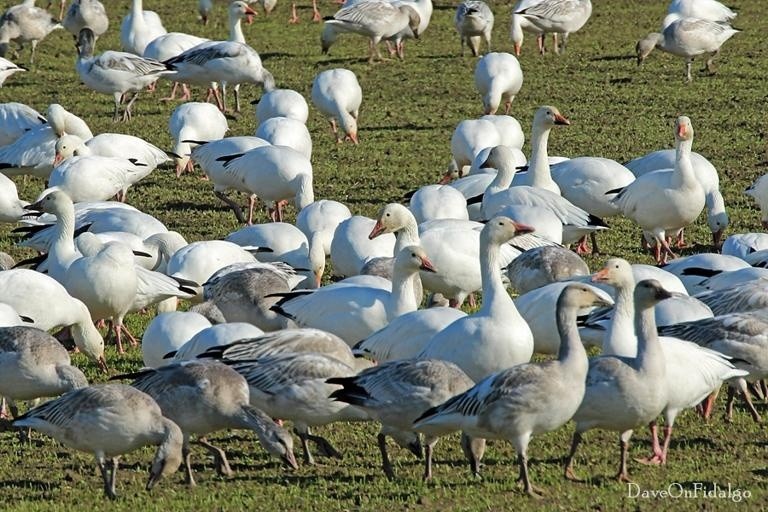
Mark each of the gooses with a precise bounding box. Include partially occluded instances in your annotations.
[0,0,768,502]
